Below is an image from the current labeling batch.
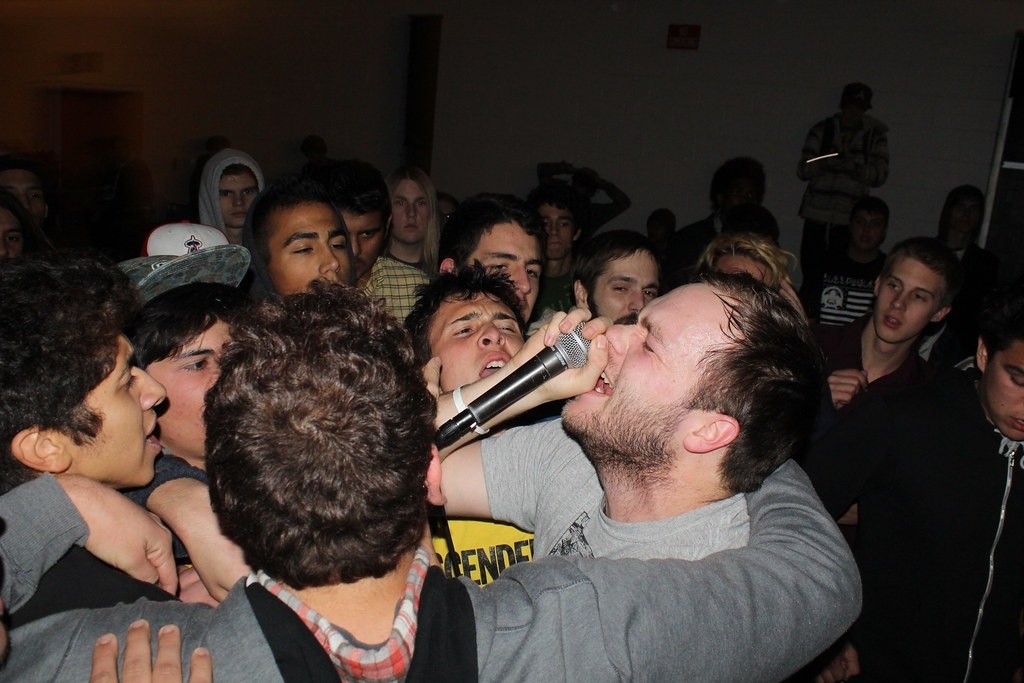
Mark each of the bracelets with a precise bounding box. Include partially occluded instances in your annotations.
[452,385,490,435]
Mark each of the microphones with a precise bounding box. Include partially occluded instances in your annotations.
[435,322,593,452]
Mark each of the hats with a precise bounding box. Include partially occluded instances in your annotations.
[838,83,872,112]
[116,243,250,314]
[142,220,230,255]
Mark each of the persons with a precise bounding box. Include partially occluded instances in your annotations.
[0,132,1024,683]
[796,82,892,317]
